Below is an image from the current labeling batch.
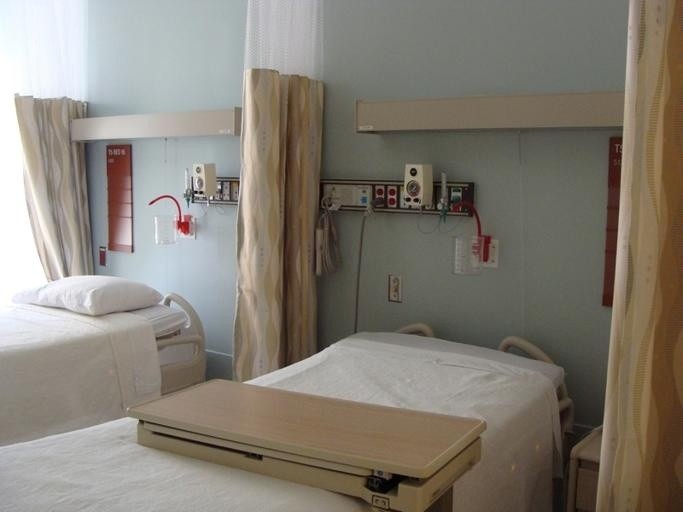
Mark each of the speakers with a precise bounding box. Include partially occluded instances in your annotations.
[192,162,217,197]
[403,163,433,207]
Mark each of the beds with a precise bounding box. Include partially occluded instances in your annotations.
[0,323,576,512]
[0,293,208,448]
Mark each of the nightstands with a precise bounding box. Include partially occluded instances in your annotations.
[565,423,601,512]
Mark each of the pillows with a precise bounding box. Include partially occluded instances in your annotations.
[11,274,166,319]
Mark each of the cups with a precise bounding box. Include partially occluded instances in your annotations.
[152,215,176,245]
[452,234,485,276]
